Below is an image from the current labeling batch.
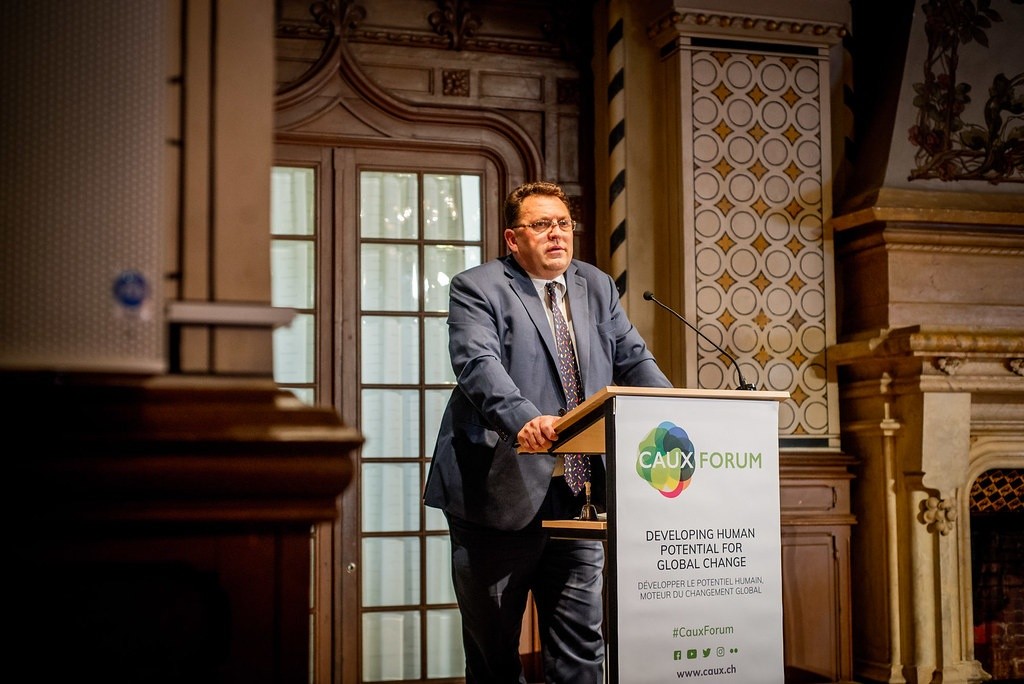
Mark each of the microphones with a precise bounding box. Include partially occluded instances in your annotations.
[644,291,757,391]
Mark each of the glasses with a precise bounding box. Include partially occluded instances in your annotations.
[509,219,577,233]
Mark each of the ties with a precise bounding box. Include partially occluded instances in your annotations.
[546,282,591,495]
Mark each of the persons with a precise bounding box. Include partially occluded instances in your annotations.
[422,181,676,684]
